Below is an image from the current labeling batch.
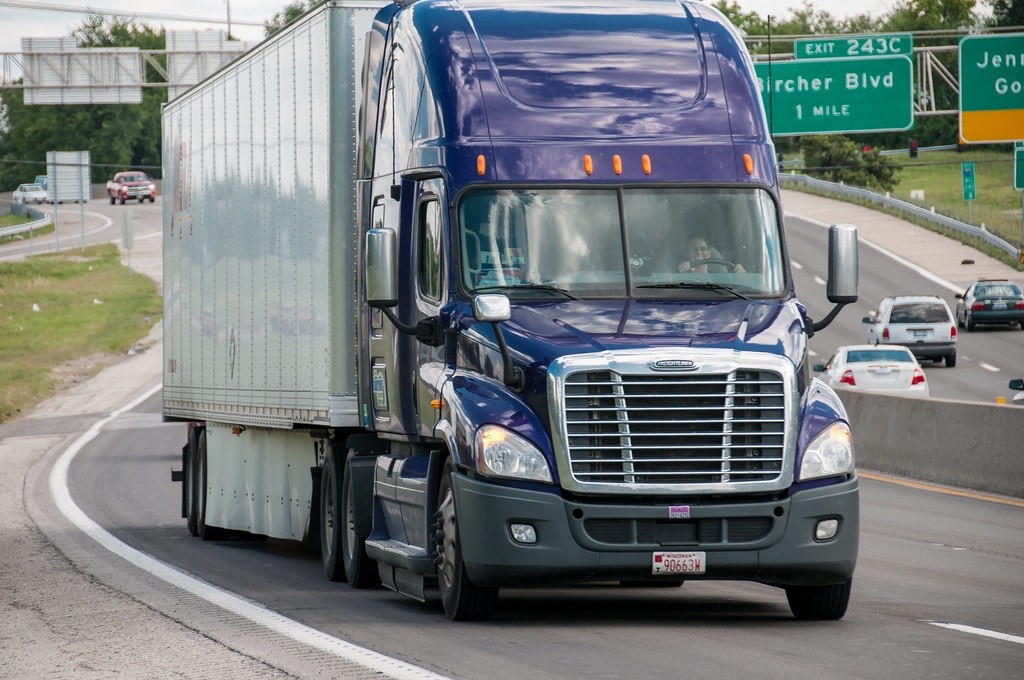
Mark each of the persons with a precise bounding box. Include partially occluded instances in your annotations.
[677,234,748,274]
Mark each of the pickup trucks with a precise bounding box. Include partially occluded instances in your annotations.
[106,172,156,204]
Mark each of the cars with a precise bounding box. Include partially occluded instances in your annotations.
[1009,378,1024,406]
[13,184,50,205]
[813,345,931,401]
[953,277,1024,331]
[35,176,48,189]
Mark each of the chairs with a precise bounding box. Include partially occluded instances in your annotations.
[708,245,728,273]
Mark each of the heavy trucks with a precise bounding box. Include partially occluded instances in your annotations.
[159,0,861,619]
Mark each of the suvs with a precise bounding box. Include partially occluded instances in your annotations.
[862,295,959,368]
